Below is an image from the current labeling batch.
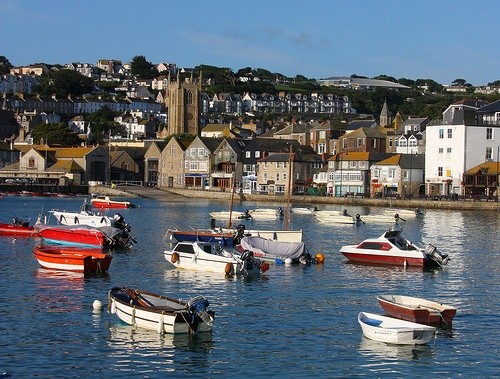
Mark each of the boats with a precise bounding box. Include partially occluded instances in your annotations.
[340,225,451,273]
[31,237,113,275]
[383,207,425,218]
[358,311,439,347]
[357,212,406,224]
[316,212,365,225]
[0,197,139,253]
[106,286,216,334]
[376,293,457,330]
[163,145,326,274]
[90,195,137,210]
[309,208,353,219]
